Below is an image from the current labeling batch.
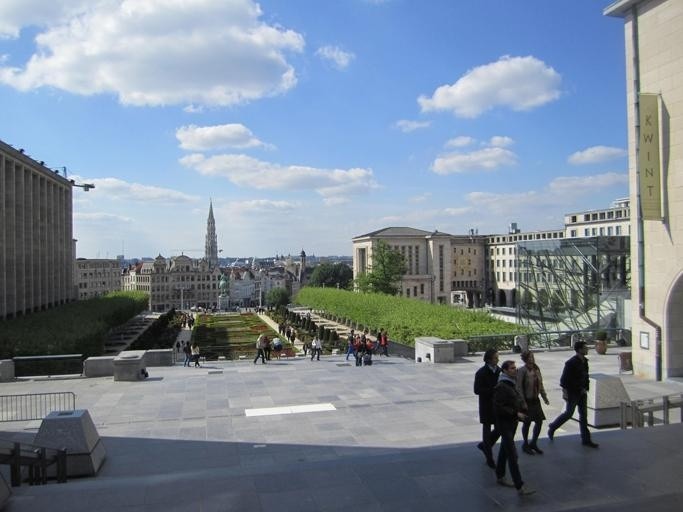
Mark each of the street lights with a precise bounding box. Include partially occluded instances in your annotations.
[175,287,184,311]
[256,281,262,307]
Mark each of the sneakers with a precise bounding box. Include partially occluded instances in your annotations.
[518,485,536,495]
[497,477,514,487]
[548,424,554,440]
[522,443,535,455]
[581,440,600,448]
[476,441,496,468]
[530,442,543,454]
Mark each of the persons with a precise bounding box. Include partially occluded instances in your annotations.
[471,348,503,469]
[515,349,551,456]
[183,341,191,367]
[548,340,602,450]
[263,337,272,360]
[310,336,322,361]
[303,342,308,355]
[345,328,390,367]
[272,336,282,360]
[252,334,266,365]
[191,343,201,367]
[493,359,539,495]
